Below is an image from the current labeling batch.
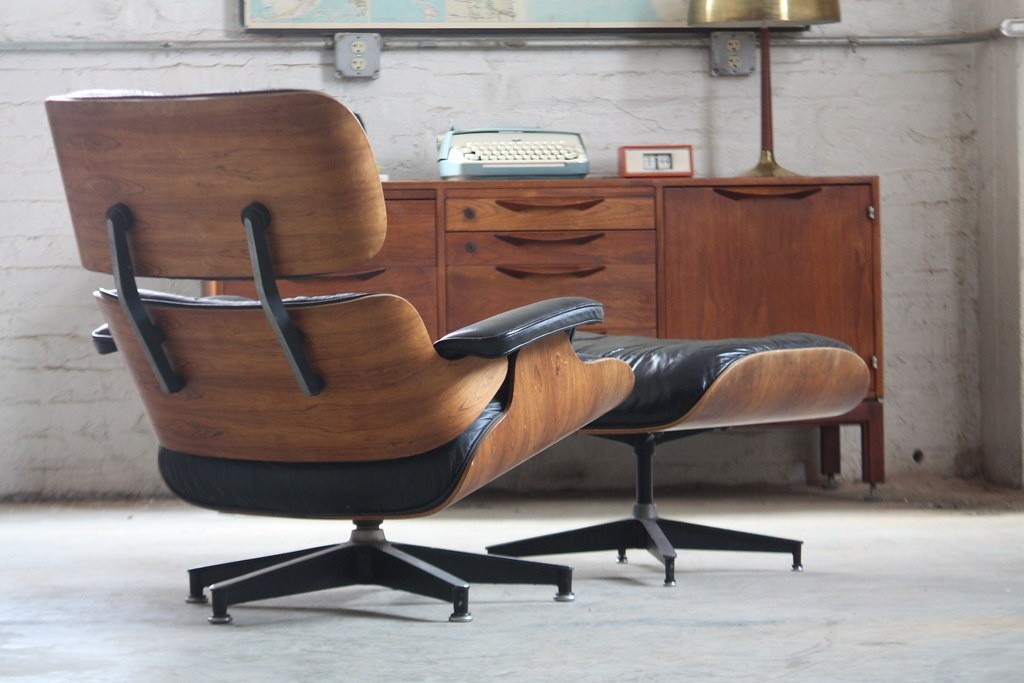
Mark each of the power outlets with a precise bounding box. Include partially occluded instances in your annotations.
[334,32,380,80]
[708,30,756,76]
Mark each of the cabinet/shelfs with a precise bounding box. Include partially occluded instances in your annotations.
[202,173,887,502]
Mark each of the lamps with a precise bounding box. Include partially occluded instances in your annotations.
[688,0,841,176]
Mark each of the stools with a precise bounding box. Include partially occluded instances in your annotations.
[484,330,871,588]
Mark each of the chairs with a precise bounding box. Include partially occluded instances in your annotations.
[44,86,635,624]
